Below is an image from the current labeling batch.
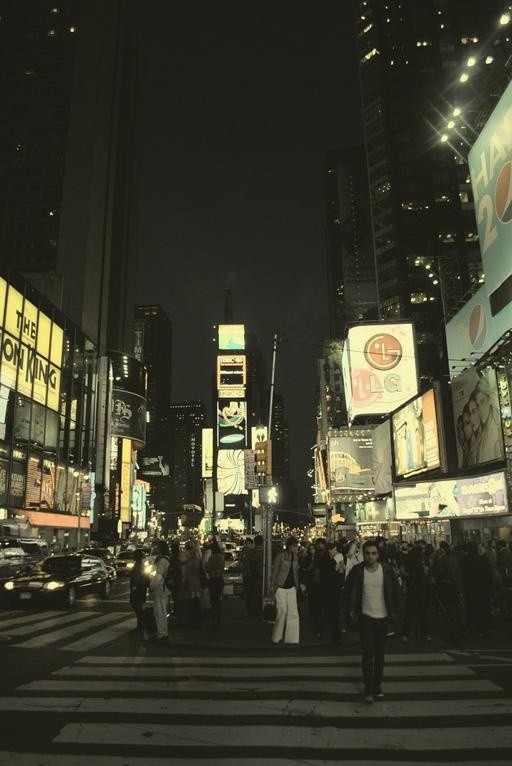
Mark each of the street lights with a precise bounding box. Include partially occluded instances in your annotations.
[73,467,92,544]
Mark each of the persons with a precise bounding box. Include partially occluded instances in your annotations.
[453,377,504,469]
[414,428,424,470]
[342,538,399,704]
[130,533,225,639]
[229,533,510,645]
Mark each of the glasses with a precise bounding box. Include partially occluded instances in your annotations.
[366,551,377,554]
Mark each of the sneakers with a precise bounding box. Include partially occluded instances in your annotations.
[365,695,374,704]
[375,689,385,697]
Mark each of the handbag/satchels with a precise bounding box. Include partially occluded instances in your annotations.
[262,596,276,623]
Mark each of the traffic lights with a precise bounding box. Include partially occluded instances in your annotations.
[260,485,280,503]
[255,439,272,484]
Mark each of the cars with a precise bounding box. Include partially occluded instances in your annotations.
[222,542,244,560]
[0,536,152,609]
